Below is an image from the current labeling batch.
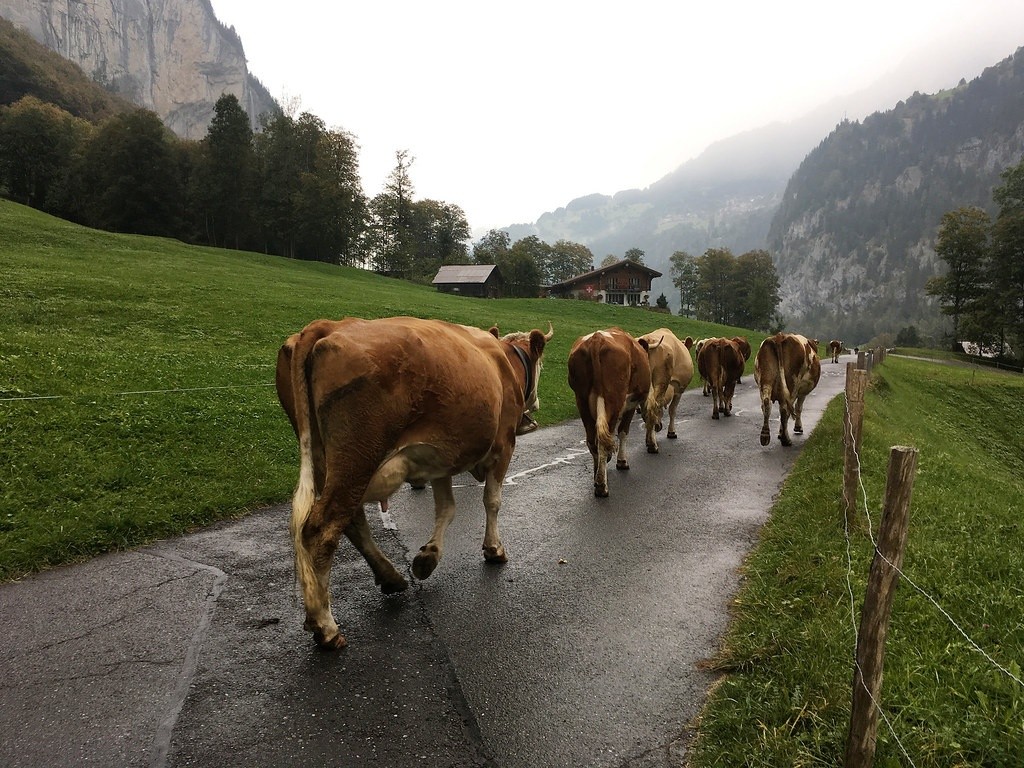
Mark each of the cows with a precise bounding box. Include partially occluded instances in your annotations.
[274,314,556,650]
[696,336,751,421]
[754,333,844,447]
[569,328,698,497]
